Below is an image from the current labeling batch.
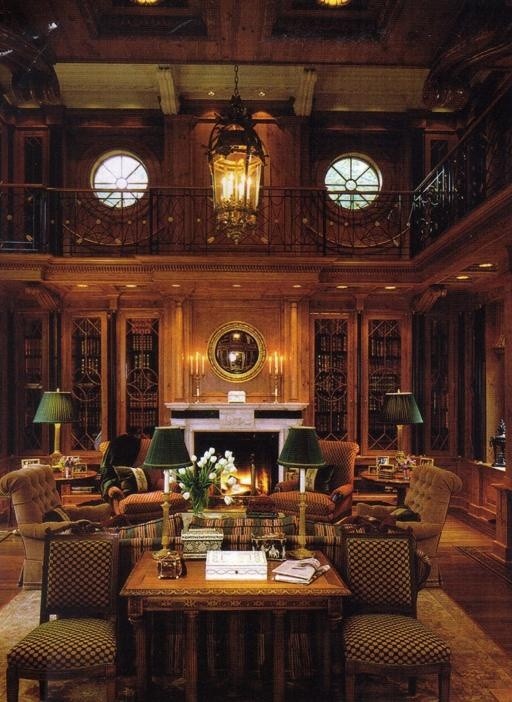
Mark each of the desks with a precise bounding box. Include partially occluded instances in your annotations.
[119,548,350,701]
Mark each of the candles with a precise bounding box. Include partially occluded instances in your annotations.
[190,356,193,375]
[281,358,283,374]
[268,357,271,376]
[196,351,199,376]
[274,351,279,375]
[201,356,205,376]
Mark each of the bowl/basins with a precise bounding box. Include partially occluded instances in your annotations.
[228,391,246,402]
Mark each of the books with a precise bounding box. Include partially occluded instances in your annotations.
[271,558,320,581]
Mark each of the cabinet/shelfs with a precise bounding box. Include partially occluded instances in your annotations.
[358,307,413,459]
[10,306,53,458]
[305,307,358,445]
[418,307,461,465]
[113,306,169,438]
[56,306,111,451]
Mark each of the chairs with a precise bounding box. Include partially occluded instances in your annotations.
[1,431,463,589]
[5,527,121,701]
[335,524,451,701]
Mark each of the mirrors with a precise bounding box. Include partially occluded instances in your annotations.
[205,321,269,384]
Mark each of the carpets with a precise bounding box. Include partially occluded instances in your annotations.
[1,578,512,701]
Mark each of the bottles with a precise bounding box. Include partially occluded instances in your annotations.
[157,552,182,579]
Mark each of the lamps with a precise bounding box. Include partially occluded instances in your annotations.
[274,425,327,559]
[377,386,426,463]
[143,425,193,560]
[32,386,79,456]
[203,1,270,246]
